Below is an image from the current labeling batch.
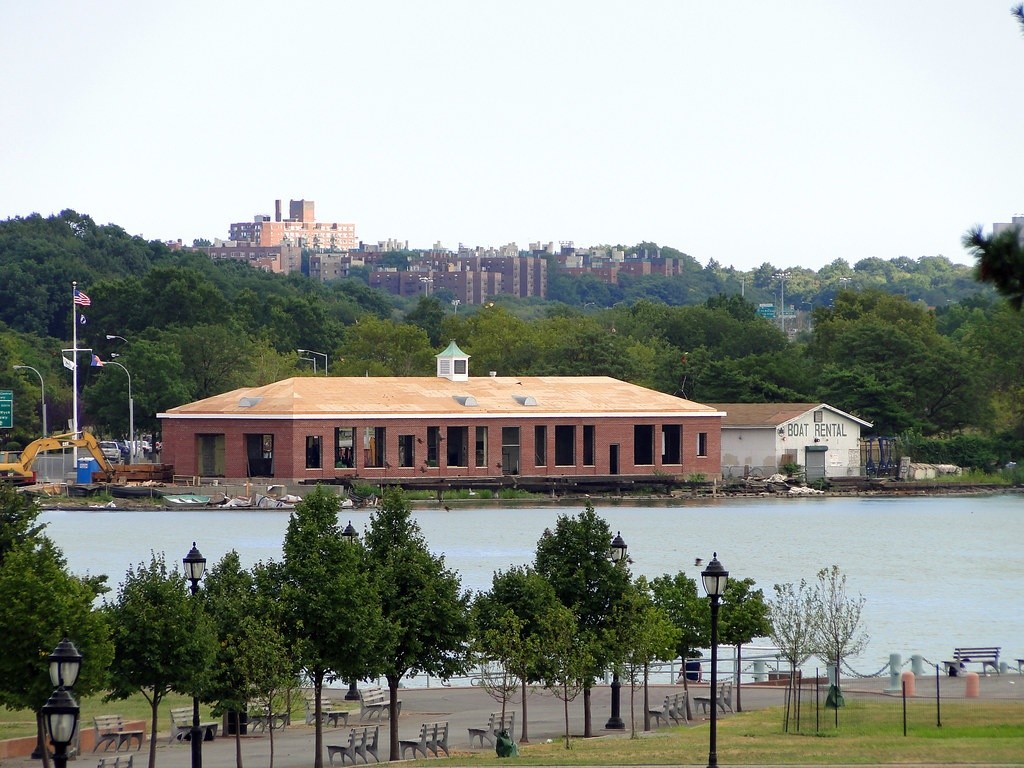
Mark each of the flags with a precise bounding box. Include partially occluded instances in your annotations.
[74,289,91,305]
[63,357,77,370]
[91,354,103,366]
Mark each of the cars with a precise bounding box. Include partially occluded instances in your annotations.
[123,432,163,458]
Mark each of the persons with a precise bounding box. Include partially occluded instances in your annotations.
[676,654,702,684]
[336,460,343,468]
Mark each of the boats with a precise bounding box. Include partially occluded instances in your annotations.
[162,494,212,510]
[64,484,100,499]
[110,485,162,500]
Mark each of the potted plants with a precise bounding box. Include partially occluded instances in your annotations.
[781,462,801,477]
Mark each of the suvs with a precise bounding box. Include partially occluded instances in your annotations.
[111,437,131,460]
[99,440,122,464]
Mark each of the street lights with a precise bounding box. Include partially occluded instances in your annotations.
[182,542,206,768]
[41,630,83,768]
[604,531,626,730]
[700,551,730,768]
[802,301,812,312]
[100,361,133,465]
[12,365,49,484]
[297,349,328,377]
[340,519,362,700]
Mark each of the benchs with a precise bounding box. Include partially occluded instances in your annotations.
[649,691,688,728]
[398,721,450,759]
[358,685,402,722]
[97,754,133,768]
[246,697,288,733]
[466,710,516,749]
[304,698,349,728]
[168,707,219,744]
[92,715,144,754]
[693,681,736,716]
[942,646,1002,677]
[1014,658,1024,674]
[327,725,380,766]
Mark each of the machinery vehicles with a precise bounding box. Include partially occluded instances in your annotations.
[0,428,128,488]
